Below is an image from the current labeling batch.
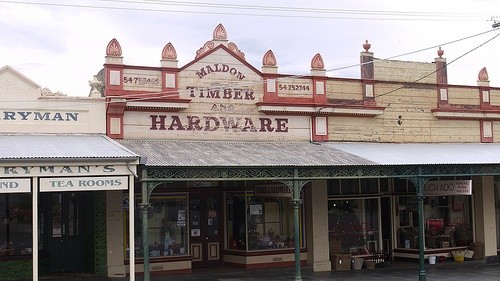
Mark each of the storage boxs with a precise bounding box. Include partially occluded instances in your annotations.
[332,253,352,271]
